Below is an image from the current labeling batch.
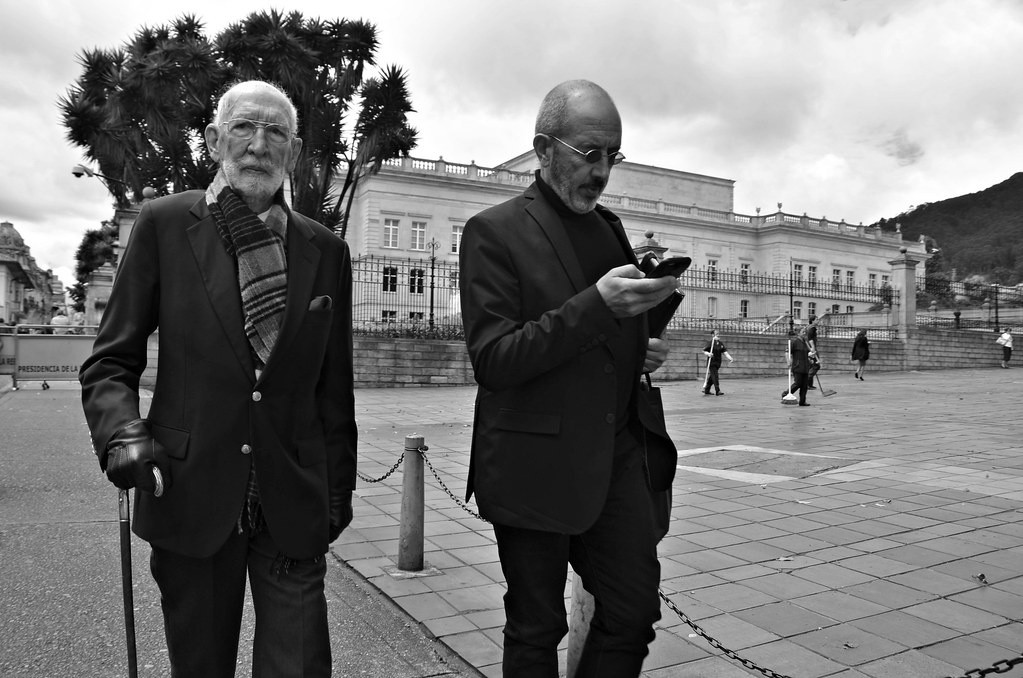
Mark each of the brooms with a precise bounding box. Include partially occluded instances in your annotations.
[781,339,798,405]
[702,336,715,392]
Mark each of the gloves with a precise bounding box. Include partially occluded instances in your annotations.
[105,419,173,493]
[329,490,354,544]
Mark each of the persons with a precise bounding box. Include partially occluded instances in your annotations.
[460,79,679,678]
[780,326,817,406]
[702,329,734,396]
[75,79,359,676]
[1000,327,1013,368]
[850,328,870,382]
[805,316,821,389]
[0,302,85,335]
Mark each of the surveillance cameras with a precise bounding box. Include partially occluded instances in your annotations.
[72,166,85,178]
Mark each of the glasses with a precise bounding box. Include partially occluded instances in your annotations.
[219,118,297,144]
[547,133,625,165]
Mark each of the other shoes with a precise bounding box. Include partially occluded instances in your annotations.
[860,377,864,381]
[800,402,810,406]
[1001,363,1009,368]
[716,391,724,396]
[705,391,714,395]
[855,372,858,378]
[807,385,817,390]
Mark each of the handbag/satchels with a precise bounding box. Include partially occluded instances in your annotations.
[996,337,1008,346]
[639,371,678,544]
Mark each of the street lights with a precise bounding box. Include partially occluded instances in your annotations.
[72,163,134,210]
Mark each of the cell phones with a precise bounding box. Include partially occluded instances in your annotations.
[645,257,693,280]
